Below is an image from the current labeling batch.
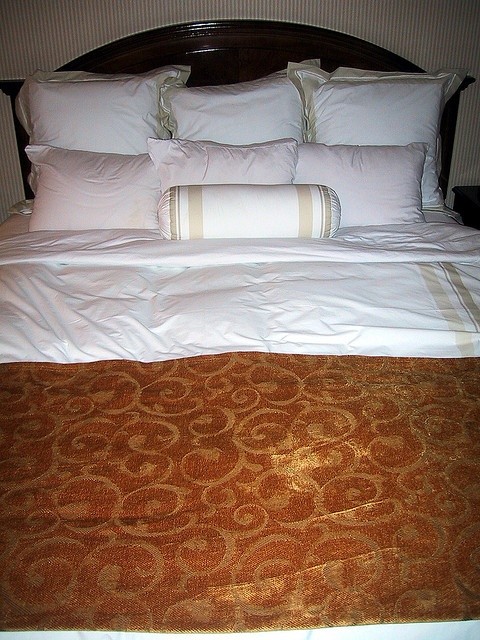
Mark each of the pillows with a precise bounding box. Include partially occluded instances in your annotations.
[147,137,297,184]
[25,145,163,231]
[293,143,430,226]
[14,63,193,155]
[157,58,321,145]
[286,61,469,212]
[155,184,341,241]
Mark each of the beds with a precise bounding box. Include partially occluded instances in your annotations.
[0,17,480,639]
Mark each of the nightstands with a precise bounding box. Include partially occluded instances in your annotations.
[451,184,479,229]
